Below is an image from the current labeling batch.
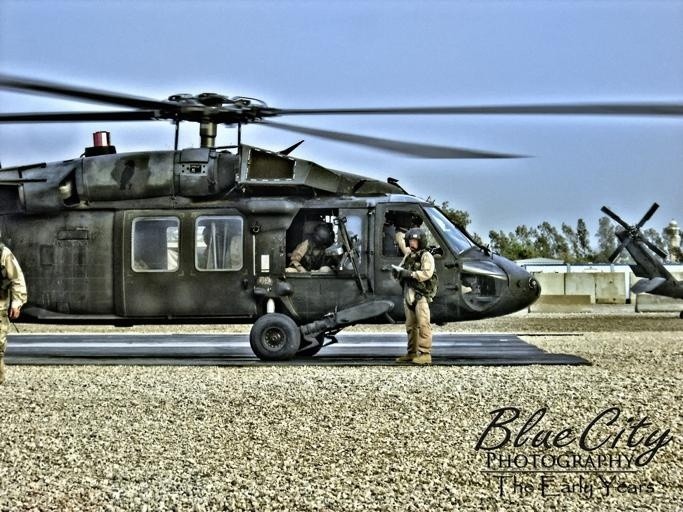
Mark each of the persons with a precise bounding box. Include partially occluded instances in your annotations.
[395,226,438,366]
[291,222,335,274]
[200,226,242,269]
[0,243,28,386]
[395,214,436,257]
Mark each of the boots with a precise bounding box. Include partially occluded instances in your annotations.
[396,353,432,364]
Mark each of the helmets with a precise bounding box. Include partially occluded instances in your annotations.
[312,225,335,250]
[405,228,428,249]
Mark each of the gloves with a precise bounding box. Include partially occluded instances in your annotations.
[392,268,412,280]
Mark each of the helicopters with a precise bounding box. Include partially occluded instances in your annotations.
[0,75,683,360]
